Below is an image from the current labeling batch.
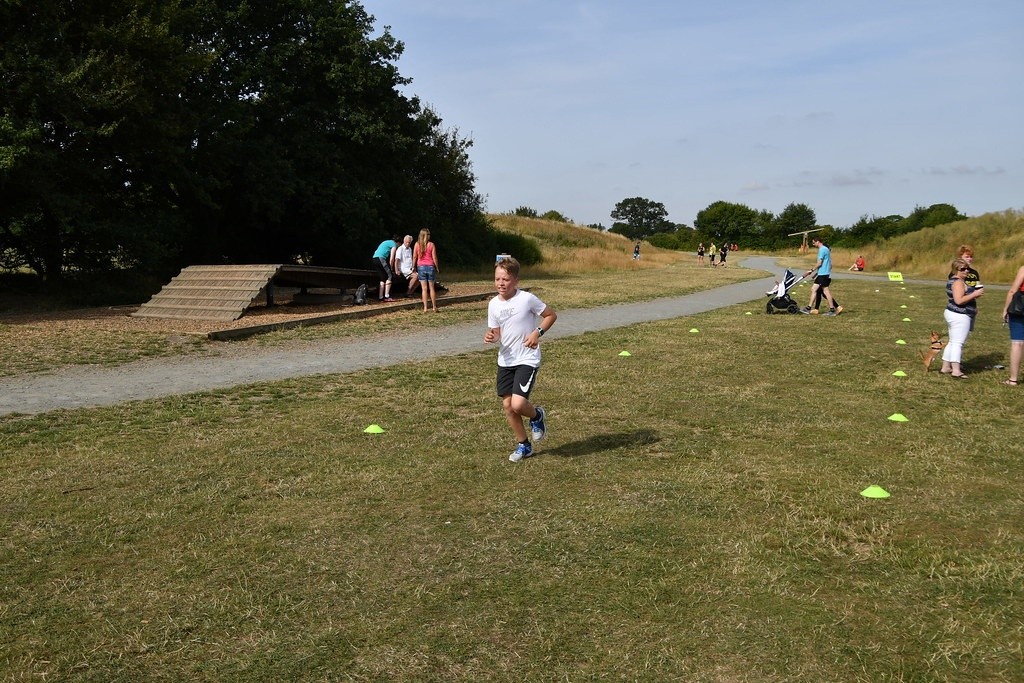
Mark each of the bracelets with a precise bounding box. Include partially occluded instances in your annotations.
[537,327,544,336]
[811,269,814,272]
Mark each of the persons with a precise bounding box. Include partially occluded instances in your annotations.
[1001,265,1024,388]
[372,234,400,301]
[799,237,843,316]
[395,235,420,297]
[411,229,439,313]
[772,282,779,291]
[940,258,984,379]
[697,243,704,265]
[483,258,557,462]
[847,255,864,272]
[716,242,728,268]
[948,245,980,290]
[708,242,716,267]
[632,242,640,261]
[729,243,738,251]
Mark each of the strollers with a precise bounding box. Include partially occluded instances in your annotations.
[766,269,812,315]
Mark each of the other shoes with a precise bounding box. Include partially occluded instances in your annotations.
[810,309,819,314]
[835,306,843,314]
[379,296,397,302]
[823,311,836,316]
[799,307,810,315]
[405,293,414,298]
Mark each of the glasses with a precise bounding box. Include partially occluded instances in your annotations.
[427,234,432,236]
[958,267,969,271]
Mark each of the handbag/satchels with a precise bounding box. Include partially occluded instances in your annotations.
[1007,291,1024,318]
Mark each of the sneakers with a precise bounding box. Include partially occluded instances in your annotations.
[529,407,546,442]
[509,443,533,462]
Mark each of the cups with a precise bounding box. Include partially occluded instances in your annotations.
[975,285,983,290]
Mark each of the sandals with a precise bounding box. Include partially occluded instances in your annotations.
[1003,378,1017,386]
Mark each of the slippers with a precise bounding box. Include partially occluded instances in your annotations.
[939,369,953,374]
[951,373,968,380]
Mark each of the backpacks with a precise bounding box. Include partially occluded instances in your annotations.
[354,284,368,305]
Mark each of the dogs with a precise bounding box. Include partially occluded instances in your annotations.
[919,329,947,372]
